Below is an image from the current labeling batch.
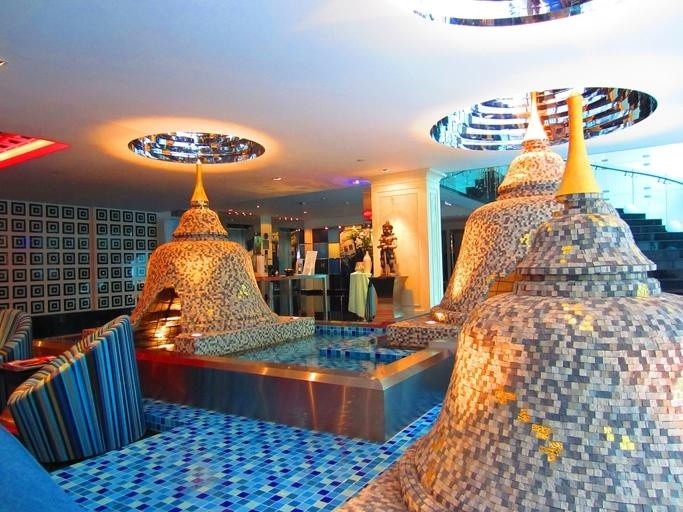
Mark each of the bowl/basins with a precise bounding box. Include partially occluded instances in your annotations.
[285,269,293,272]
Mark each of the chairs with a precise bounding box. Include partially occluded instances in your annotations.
[0,309,33,372]
[10,316,146,468]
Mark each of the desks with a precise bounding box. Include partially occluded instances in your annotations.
[258,275,326,321]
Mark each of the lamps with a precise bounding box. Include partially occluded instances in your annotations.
[0,134,66,170]
[427,84,658,152]
[129,132,266,165]
[407,0,599,28]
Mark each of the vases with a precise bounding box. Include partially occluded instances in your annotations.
[363,251,372,273]
